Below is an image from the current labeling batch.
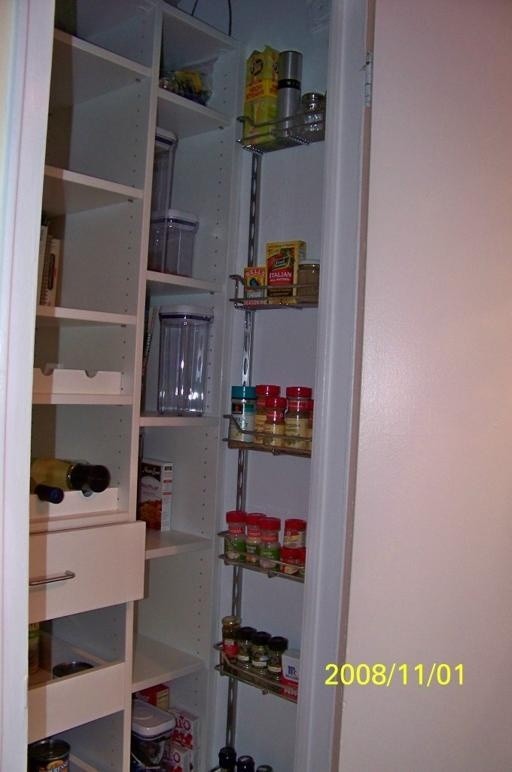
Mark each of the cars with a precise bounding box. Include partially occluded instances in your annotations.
[157,304,214,416]
[301,89,326,138]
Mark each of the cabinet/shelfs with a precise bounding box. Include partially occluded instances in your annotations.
[30,456,110,504]
[275,48,302,137]
[295,260,320,305]
[28,0,329,771]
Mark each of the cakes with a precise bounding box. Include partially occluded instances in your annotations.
[27,738,71,772]
[294,261,320,304]
[53,662,94,680]
[221,615,289,690]
[296,94,326,141]
[227,385,314,456]
